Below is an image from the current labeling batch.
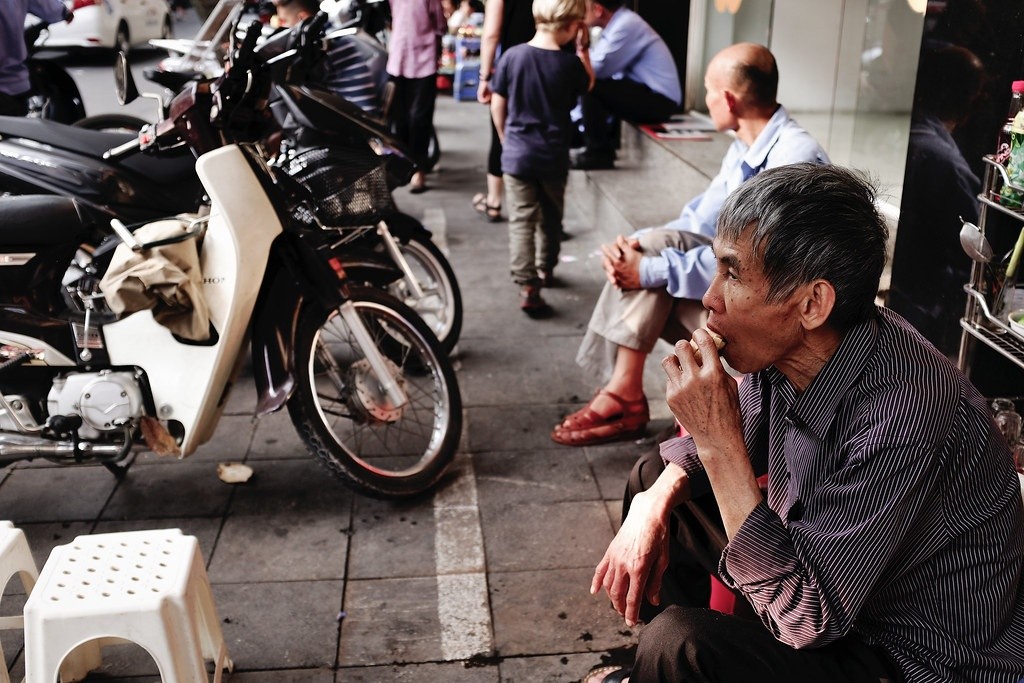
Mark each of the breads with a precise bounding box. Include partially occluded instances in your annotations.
[689,327,725,365]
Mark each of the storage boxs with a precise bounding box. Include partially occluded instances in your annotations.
[454,36,501,101]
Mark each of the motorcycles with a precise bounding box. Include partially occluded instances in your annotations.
[0,0,463,502]
[0,10,464,376]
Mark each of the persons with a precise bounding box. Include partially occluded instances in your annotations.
[886,40,986,352]
[0,0,74,118]
[263,0,681,320]
[551,43,830,445]
[589,162,1024,683]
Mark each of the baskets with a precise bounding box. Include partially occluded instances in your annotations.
[280,146,394,226]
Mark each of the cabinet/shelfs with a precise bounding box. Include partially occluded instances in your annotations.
[957,156,1024,485]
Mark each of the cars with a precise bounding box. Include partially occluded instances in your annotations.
[24,1,174,64]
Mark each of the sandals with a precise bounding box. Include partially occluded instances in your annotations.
[472,194,501,222]
[581,666,632,683]
[551,390,650,445]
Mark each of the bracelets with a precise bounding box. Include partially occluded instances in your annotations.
[479,72,491,81]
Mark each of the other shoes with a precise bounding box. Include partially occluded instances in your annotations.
[569,152,614,170]
[411,184,422,193]
[538,270,553,285]
[519,287,546,314]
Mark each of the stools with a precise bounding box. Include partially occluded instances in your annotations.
[0,519,41,683]
[21,527,234,683]
